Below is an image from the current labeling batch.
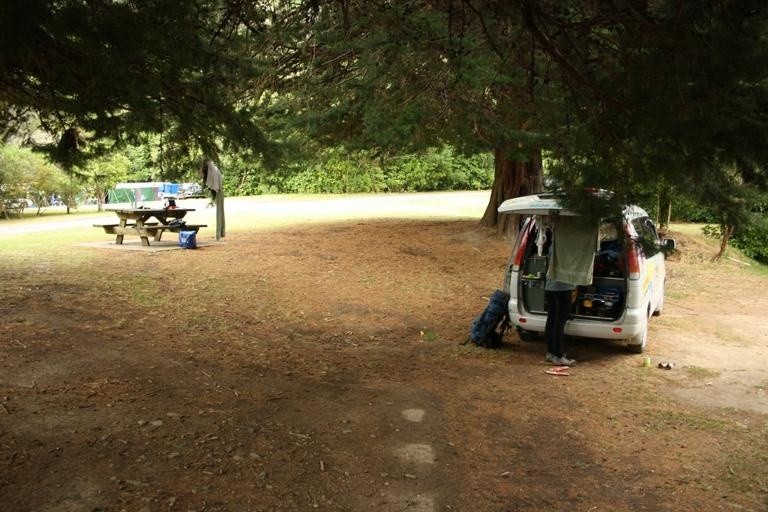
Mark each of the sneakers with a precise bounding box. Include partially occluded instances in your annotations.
[547,352,576,366]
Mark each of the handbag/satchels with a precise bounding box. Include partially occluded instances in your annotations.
[472,290,510,348]
[179,230,196,249]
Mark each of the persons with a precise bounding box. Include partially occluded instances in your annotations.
[542,242,578,368]
[48,189,109,207]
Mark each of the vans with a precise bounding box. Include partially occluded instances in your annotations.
[495,186,676,353]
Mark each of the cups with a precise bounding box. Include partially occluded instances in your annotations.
[642,356,651,367]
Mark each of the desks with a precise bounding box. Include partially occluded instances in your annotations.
[104,207,196,247]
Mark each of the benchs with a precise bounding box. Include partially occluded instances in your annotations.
[90,221,208,247]
